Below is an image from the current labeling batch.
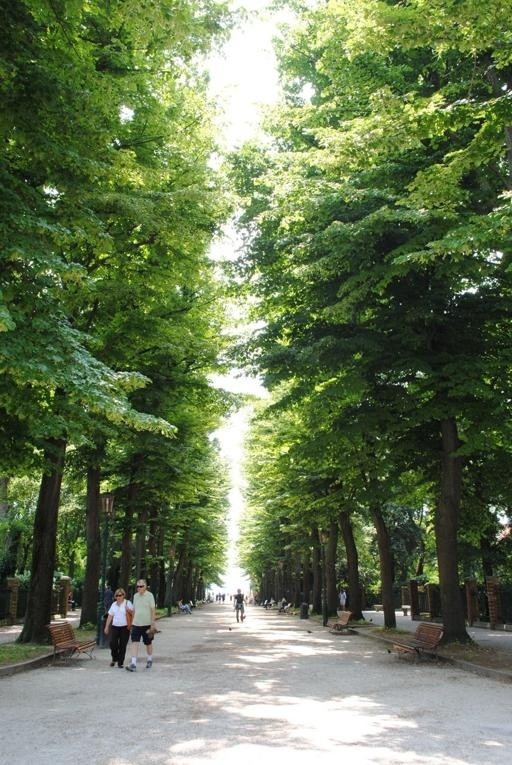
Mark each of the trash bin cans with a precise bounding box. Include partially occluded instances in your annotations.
[96,614,112,649]
[300,602,308,619]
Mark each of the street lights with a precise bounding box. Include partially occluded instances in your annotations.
[319,528,328,628]
[168,542,178,617]
[96,490,115,648]
[277,559,284,600]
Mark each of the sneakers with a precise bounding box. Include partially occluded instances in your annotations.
[110,662,115,666]
[126,663,136,672]
[147,661,152,668]
[119,665,123,668]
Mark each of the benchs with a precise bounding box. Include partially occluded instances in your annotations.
[327,611,353,635]
[391,622,448,666]
[45,622,96,667]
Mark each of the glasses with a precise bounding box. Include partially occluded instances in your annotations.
[115,594,122,597]
[137,586,143,589]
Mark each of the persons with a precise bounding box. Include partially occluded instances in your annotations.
[338,590,348,611]
[179,598,192,615]
[103,586,114,611]
[231,589,247,624]
[187,599,194,610]
[103,587,133,669]
[125,578,157,672]
[206,592,226,605]
[264,596,274,610]
[277,597,288,613]
